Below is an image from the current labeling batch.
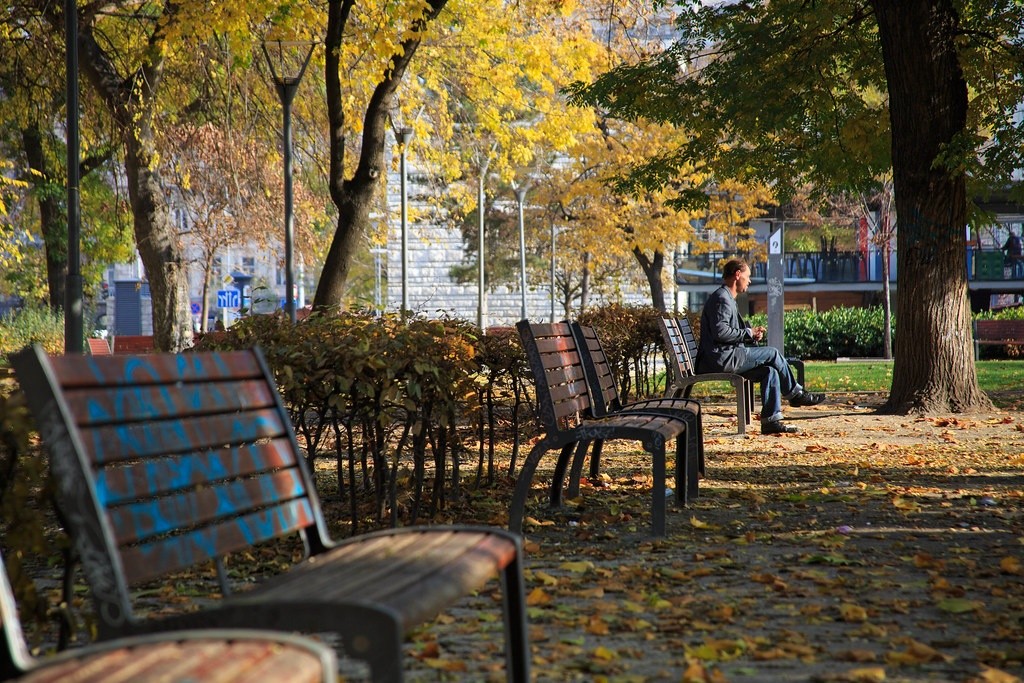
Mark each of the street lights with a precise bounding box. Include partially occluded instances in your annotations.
[446,122,531,336]
[231,270,253,318]
[258,36,323,330]
[501,172,544,322]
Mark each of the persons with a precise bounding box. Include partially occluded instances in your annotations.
[695,260,826,434]
[215,319,224,331]
[1001,232,1021,266]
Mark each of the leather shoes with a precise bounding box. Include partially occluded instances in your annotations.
[790,389,826,407]
[761,422,797,433]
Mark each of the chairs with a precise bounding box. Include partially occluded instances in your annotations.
[0,555,340,683]
[658,315,754,434]
[567,321,704,500]
[508,319,689,536]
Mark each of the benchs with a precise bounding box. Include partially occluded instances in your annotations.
[5,342,532,683]
[973,320,1024,361]
[110,336,155,353]
[86,338,110,354]
